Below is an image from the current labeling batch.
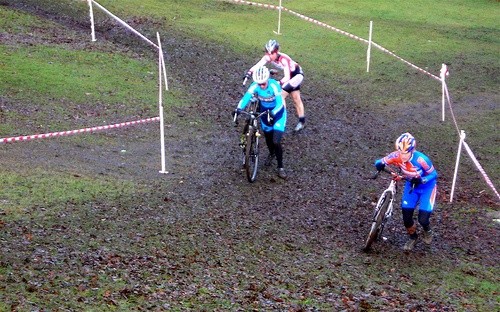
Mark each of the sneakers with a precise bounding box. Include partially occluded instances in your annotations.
[422,230,434,245]
[403,235,419,251]
[277,168,288,178]
[292,121,308,132]
[265,153,276,165]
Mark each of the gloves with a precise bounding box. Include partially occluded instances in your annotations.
[266,113,274,125]
[243,74,251,81]
[376,162,386,173]
[410,177,423,189]
[231,110,241,120]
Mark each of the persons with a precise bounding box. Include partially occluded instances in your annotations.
[231,65,287,178]
[375,133,437,252]
[241,39,307,133]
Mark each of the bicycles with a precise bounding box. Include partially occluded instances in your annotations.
[363,162,418,251]
[233,97,270,183]
[242,68,278,88]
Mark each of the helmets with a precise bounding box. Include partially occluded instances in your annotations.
[265,40,279,54]
[395,132,416,154]
[252,65,270,84]
